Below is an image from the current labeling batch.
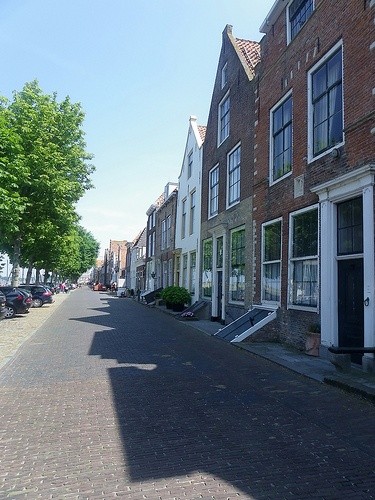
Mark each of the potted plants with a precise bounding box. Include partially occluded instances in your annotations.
[302,324,321,356]
[166,287,192,312]
[159,286,174,309]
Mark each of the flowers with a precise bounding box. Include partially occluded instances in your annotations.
[180,312,194,317]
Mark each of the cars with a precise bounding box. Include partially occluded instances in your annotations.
[17,285,55,308]
[0,286,32,318]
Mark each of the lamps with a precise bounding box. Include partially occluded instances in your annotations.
[150,271,157,279]
